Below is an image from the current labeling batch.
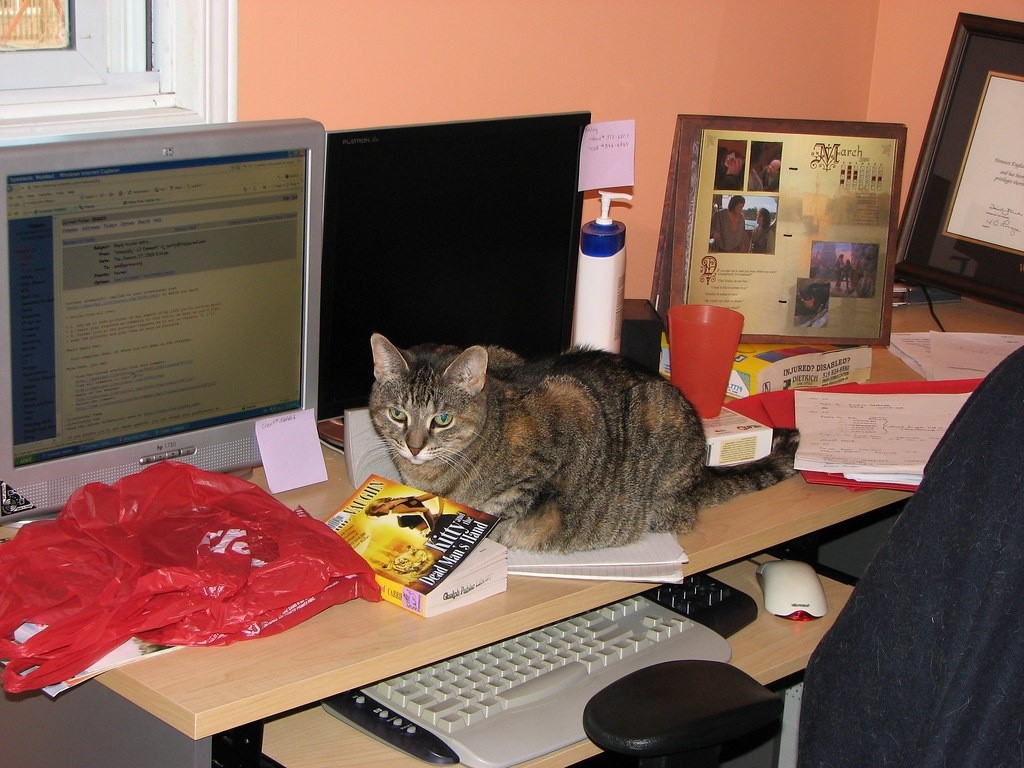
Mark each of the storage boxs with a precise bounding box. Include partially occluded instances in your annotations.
[660,330,873,399]
[699,406,773,467]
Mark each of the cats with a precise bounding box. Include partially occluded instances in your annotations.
[368,333,800,550]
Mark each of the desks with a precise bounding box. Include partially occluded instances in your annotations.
[0,294,1024,768]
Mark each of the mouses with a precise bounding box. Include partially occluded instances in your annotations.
[757,559,827,621]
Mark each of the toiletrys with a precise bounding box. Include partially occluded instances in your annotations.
[571,190,634,355]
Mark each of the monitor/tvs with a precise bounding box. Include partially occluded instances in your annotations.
[0,120,328,529]
[317,112,593,425]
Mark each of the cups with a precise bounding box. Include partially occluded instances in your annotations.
[665,303,746,420]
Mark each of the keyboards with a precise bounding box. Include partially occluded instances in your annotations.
[321,574,759,768]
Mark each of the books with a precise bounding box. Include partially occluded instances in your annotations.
[323,471,691,618]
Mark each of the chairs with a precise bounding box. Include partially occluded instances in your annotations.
[582,344,1024,768]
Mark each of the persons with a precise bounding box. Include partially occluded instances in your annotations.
[713,139,874,316]
[363,493,477,554]
